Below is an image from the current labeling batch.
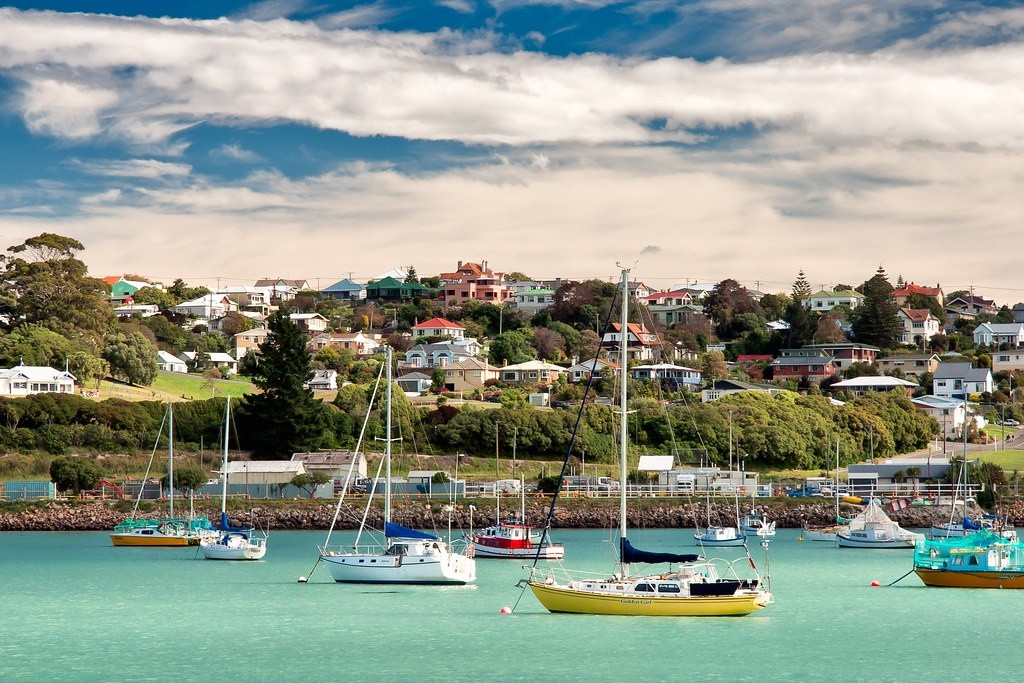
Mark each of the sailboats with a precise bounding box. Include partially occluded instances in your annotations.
[472,421,547,544]
[198,394,270,560]
[804,441,898,541]
[316,343,477,584]
[913,381,1024,590]
[514,260,776,616]
[108,400,200,547]
[835,423,926,548]
[741,477,777,537]
[693,474,747,547]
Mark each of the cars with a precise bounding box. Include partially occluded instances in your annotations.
[999,419,1020,426]
[789,486,820,498]
[834,489,850,499]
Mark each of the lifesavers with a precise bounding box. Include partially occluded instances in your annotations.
[467,544,476,555]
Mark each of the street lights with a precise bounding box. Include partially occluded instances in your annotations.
[454,452,466,508]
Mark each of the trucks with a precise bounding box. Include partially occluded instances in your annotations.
[560,476,619,491]
[335,477,372,494]
[676,474,695,496]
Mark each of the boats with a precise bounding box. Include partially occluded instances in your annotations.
[113,488,212,533]
[464,505,566,560]
[931,519,1016,538]
